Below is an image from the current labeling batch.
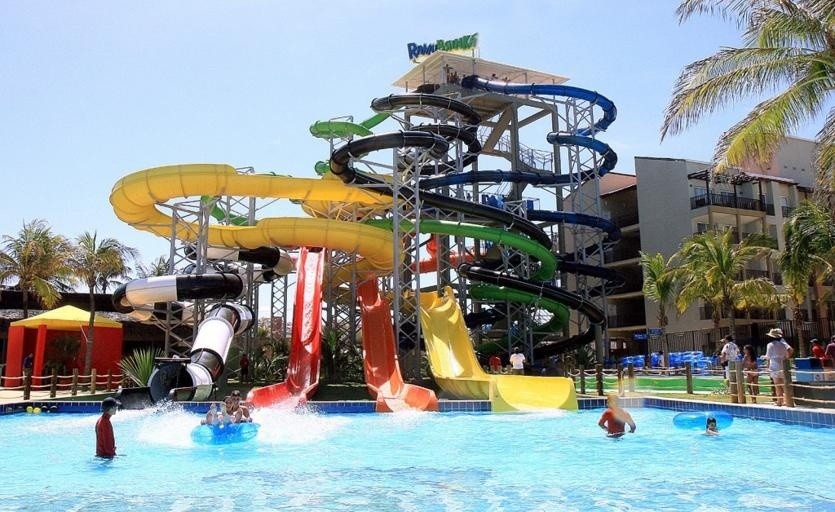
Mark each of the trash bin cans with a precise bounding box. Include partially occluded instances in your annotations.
[796,358,835,382]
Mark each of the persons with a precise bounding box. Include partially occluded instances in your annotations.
[509,347,526,375]
[657,351,666,376]
[199,389,252,424]
[713,325,795,409]
[92,400,120,458]
[598,394,636,435]
[805,334,835,370]
[489,353,504,374]
[705,417,720,436]
[23,353,62,380]
[240,354,250,384]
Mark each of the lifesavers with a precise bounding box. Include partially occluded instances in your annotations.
[191,422,260,444]
[622,351,718,368]
[674,409,733,430]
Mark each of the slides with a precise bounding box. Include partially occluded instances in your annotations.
[358,229,486,412]
[244,244,328,412]
[109,163,581,412]
[328,83,628,367]
[404,73,618,377]
[112,241,295,415]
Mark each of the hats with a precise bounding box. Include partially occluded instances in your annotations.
[725,335,735,340]
[767,327,784,339]
[103,397,121,409]
[810,338,818,342]
